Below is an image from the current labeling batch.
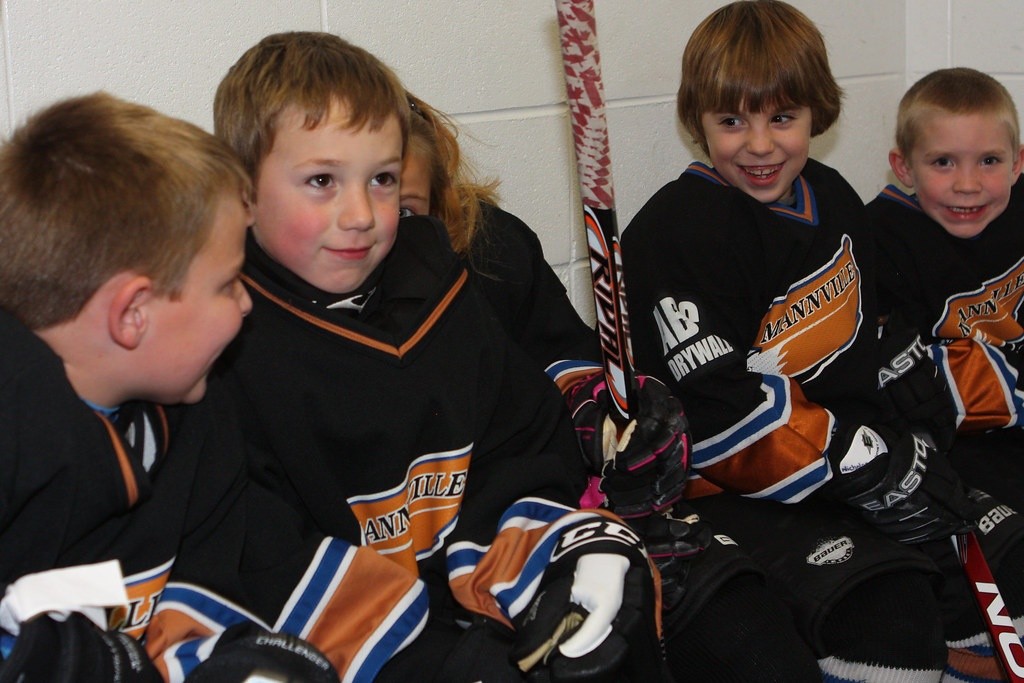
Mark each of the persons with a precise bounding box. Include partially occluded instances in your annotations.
[0,0,1024,683]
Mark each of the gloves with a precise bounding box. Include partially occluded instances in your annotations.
[845,424,975,543]
[519,527,663,683]
[542,370,714,559]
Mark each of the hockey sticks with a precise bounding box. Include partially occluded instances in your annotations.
[948,528,1024,683]
[554,0,638,438]
